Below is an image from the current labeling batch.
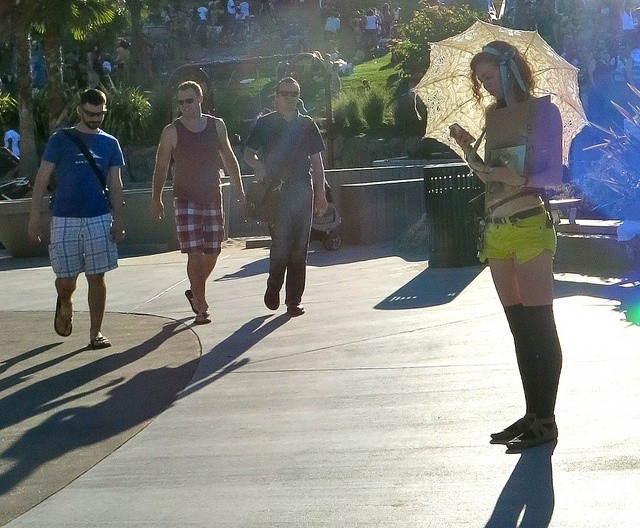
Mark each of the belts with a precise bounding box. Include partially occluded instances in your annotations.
[486,203,545,224]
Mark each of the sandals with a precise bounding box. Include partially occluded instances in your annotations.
[490,412,535,445]
[506,415,558,450]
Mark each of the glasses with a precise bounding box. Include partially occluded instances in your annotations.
[278,89,300,97]
[178,97,198,105]
[81,107,108,119]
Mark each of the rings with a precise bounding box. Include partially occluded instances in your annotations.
[504,160,510,166]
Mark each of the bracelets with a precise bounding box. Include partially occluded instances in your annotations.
[520,175,528,186]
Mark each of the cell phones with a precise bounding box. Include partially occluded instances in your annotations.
[449,122,476,145]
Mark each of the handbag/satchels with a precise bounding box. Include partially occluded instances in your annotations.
[61,129,124,210]
[475,190,537,252]
[242,114,311,222]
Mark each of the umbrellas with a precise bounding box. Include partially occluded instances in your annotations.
[411,17,590,176]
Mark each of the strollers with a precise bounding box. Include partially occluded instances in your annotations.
[310,168,342,251]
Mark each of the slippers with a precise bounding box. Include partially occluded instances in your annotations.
[185,290,208,314]
[54,295,73,336]
[193,312,211,324]
[88,335,111,349]
[288,306,305,317]
[264,288,280,310]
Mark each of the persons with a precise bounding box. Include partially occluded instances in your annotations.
[356,80,372,95]
[450,42,563,451]
[553,0,640,120]
[330,63,342,95]
[150,80,246,324]
[322,0,404,71]
[3,128,20,157]
[27,88,126,349]
[100,0,251,85]
[244,77,329,317]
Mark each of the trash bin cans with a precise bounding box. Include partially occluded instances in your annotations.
[424,163,489,268]
